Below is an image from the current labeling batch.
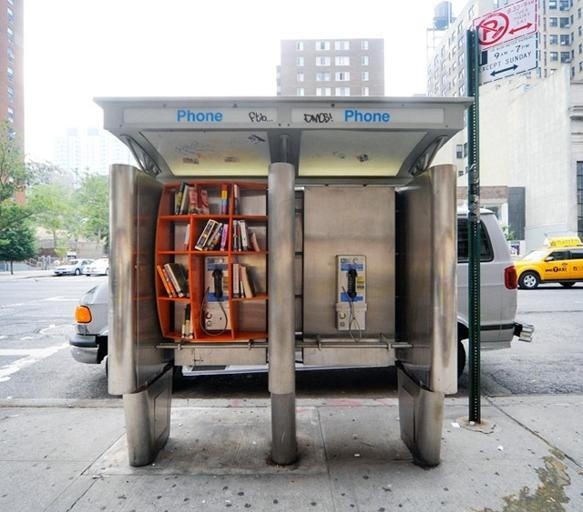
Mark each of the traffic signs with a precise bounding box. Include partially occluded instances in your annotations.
[477,30,540,87]
[472,1,539,52]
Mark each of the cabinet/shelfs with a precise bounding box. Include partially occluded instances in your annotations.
[154,181,268,343]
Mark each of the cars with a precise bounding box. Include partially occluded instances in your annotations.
[510,236,583,290]
[81,257,108,276]
[54,258,93,276]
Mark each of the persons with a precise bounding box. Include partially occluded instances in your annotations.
[542,232,551,247]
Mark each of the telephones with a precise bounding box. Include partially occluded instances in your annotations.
[347,269,357,297]
[212,270,226,298]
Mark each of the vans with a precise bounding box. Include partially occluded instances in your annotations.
[62,251,77,265]
[69,198,534,392]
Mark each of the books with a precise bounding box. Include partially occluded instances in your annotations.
[155,180,264,340]
